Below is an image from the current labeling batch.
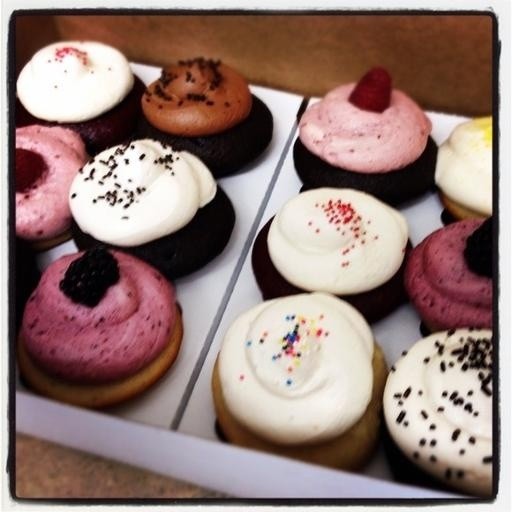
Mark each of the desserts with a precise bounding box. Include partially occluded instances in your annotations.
[211,65,493,498]
[15,41,274,411]
